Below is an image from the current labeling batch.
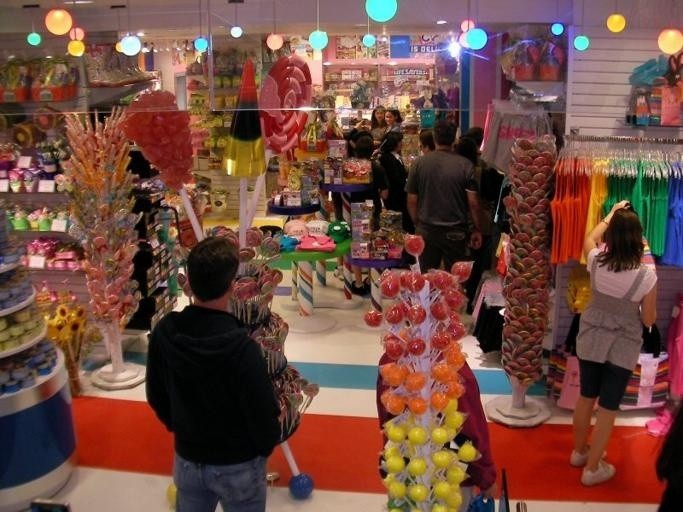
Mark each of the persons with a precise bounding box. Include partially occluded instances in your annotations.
[568,199,659,487]
[347,104,511,318]
[145,235,284,511]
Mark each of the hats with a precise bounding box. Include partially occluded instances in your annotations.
[284,219,350,244]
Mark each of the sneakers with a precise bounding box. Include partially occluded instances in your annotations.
[569,450,607,466]
[581,461,616,486]
[352,278,370,296]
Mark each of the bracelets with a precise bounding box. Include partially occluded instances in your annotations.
[600,219,608,226]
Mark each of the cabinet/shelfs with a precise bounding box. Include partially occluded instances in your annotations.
[323,59,438,111]
[184,66,266,210]
[166,261,313,512]
[0,255,77,511]
[0,81,177,348]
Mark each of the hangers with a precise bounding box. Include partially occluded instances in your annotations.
[552,133,683,181]
[490,89,547,118]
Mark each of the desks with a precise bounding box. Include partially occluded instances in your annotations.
[345,254,402,331]
[266,177,372,311]
[258,233,353,333]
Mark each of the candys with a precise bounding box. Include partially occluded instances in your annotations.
[248,55,312,229]
[503,134,557,387]
[204,223,319,440]
[48,303,86,365]
[363,236,481,512]
[64,105,144,330]
